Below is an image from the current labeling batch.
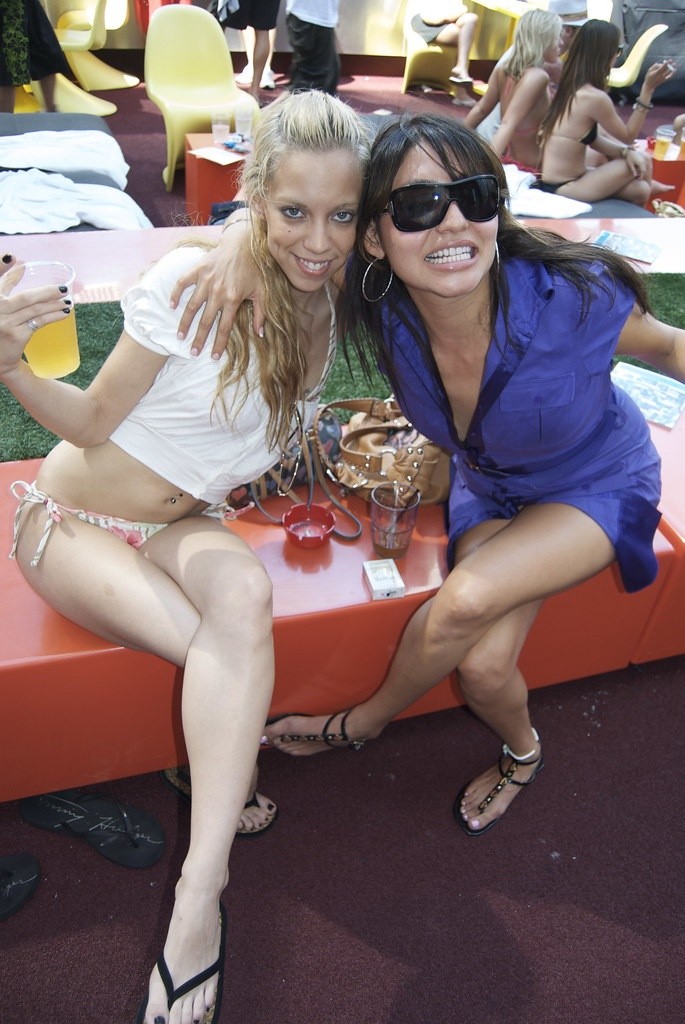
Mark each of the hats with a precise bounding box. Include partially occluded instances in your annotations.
[545,0,590,26]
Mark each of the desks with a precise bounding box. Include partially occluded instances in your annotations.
[631,138,685,215]
[184,133,246,227]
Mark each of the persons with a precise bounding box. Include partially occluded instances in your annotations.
[411,0,478,107]
[170,112,685,836]
[465,0,677,209]
[0,87,368,1024]
[191,0,340,103]
[0,0,67,113]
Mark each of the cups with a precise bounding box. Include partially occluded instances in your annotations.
[211,112,230,145]
[653,128,676,161]
[371,481,421,560]
[680,127,685,155]
[235,108,253,142]
[0,261,80,379]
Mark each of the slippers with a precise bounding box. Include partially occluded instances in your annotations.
[19,792,167,869]
[0,852,42,916]
[135,901,225,1024]
[156,761,276,836]
[450,74,475,86]
[453,97,478,108]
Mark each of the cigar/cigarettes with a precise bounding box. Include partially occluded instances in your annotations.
[663,60,676,72]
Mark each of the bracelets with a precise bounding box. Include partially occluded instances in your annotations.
[632,103,649,113]
[621,147,635,158]
[635,97,653,109]
[221,217,249,234]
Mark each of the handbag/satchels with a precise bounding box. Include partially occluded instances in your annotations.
[227,406,342,514]
[314,391,452,505]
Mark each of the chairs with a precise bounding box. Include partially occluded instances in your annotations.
[2,1,669,235]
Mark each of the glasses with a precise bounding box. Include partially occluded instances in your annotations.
[381,173,501,233]
[615,45,624,59]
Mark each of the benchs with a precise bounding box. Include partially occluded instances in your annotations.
[2,424,685,804]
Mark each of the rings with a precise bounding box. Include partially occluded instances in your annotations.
[27,319,37,330]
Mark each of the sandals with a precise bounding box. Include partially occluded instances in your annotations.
[261,714,375,758]
[451,730,544,834]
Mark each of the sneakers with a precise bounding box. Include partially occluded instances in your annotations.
[236,63,254,85]
[259,67,276,90]
